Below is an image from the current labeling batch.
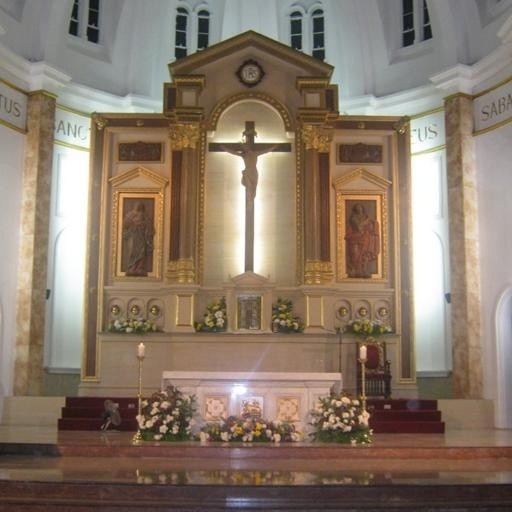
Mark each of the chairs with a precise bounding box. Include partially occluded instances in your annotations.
[356,341,391,401]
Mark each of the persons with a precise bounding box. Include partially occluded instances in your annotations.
[344,202,384,277]
[121,201,156,276]
[220,140,277,208]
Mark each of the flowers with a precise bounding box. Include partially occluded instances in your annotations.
[337,318,392,336]
[272,296,305,334]
[200,414,304,444]
[193,296,226,332]
[107,317,162,334]
[137,385,195,441]
[310,388,370,447]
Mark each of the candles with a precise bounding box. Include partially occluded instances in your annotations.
[360,345,367,359]
[138,345,145,357]
